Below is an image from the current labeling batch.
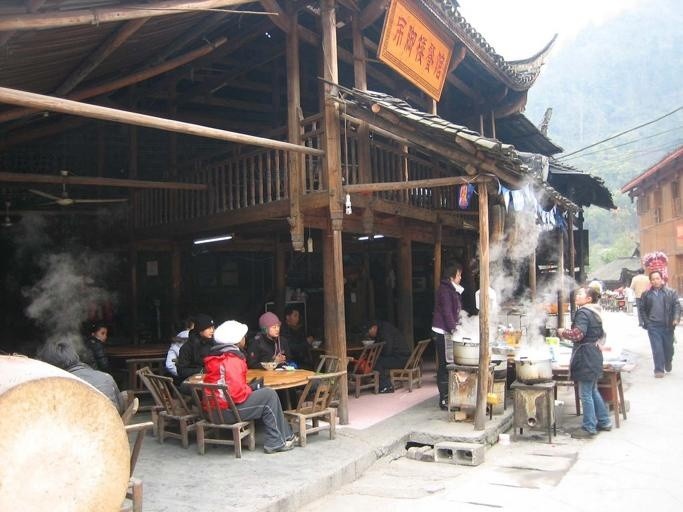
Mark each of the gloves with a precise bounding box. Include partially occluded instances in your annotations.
[247,375,265,393]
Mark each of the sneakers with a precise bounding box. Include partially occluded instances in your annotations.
[597,424,613,431]
[570,427,599,440]
[653,360,675,379]
[378,385,395,395]
[263,432,302,455]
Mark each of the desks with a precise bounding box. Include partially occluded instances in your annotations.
[546,358,641,430]
[182,367,315,434]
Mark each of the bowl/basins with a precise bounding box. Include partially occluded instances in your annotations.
[260,362,278,371]
[361,341,375,346]
[312,341,323,348]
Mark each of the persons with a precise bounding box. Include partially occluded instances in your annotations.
[78,324,113,378]
[557,269,681,438]
[41,332,126,418]
[165,304,410,453]
[430,264,465,411]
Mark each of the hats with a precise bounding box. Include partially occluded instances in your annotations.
[191,309,216,333]
[258,310,282,332]
[212,319,250,346]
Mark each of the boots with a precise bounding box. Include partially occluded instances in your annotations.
[437,368,461,412]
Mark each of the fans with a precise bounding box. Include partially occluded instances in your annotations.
[24,169,131,211]
[0,199,20,231]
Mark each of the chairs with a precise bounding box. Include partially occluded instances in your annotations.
[137,367,187,437]
[189,380,257,458]
[283,371,347,447]
[293,354,341,409]
[391,338,432,391]
[125,358,168,417]
[143,374,203,450]
[348,341,387,398]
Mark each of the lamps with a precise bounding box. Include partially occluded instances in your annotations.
[341,192,355,217]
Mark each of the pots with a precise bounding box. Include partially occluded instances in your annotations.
[452,337,493,365]
[515,355,554,385]
